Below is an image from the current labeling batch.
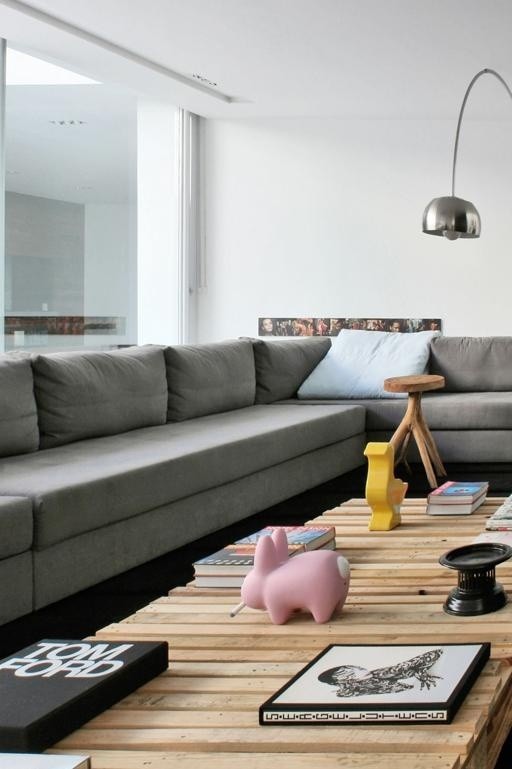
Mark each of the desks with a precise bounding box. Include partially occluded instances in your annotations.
[382,372,447,489]
[42,492,512,768]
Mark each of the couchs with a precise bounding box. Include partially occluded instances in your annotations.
[1,333,511,627]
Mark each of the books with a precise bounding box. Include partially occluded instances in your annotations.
[485,494,512,531]
[258,642,491,725]
[426,481,489,516]
[192,526,336,588]
[0,639,169,769]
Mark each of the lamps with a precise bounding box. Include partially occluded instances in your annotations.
[422,68,512,241]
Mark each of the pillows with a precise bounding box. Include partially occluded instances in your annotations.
[296,329,441,400]
